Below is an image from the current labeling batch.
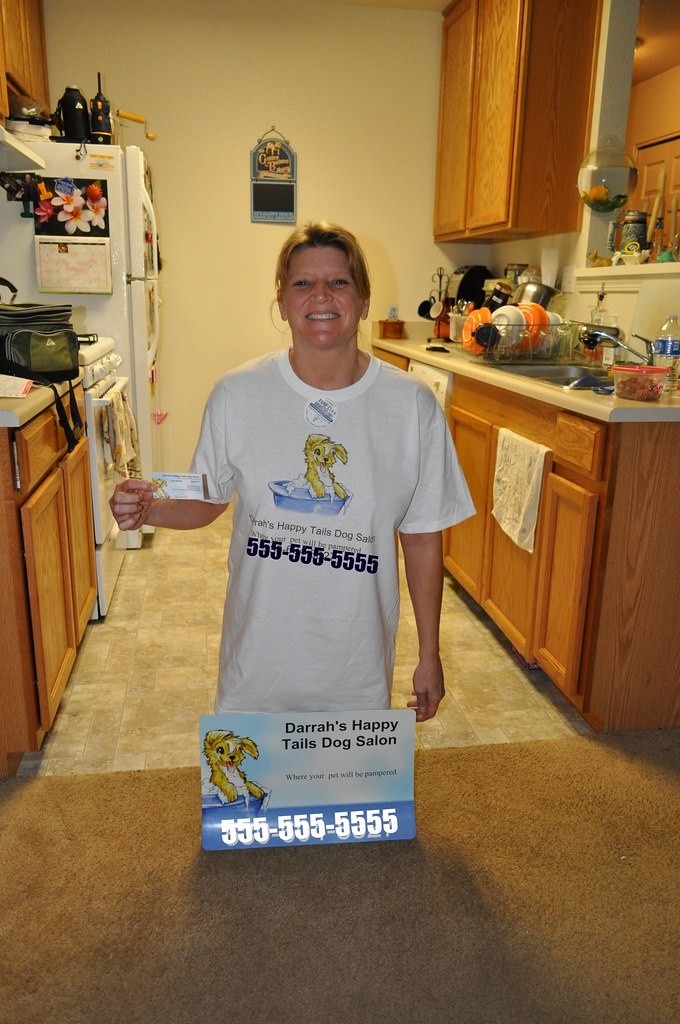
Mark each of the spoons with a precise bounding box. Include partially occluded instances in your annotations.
[458,299,468,313]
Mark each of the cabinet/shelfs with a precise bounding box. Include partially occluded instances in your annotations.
[0,0,52,120]
[441,373,680,733]
[0,383,98,778]
[431,0,604,247]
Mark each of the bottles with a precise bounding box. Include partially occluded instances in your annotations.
[483,282,512,313]
[652,314,680,400]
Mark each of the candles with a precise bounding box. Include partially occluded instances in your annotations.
[670,195,678,241]
[659,169,667,217]
[646,192,661,242]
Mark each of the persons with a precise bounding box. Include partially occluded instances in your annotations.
[11,174,36,201]
[109,220,476,722]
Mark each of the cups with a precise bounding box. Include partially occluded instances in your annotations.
[430,296,451,324]
[540,248,559,289]
[447,312,467,342]
[418,296,437,321]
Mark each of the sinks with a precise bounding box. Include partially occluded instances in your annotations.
[537,378,614,389]
[471,361,610,378]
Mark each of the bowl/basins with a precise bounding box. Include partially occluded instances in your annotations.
[612,365,671,401]
[462,301,563,356]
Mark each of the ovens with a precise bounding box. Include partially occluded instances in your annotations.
[85,368,126,620]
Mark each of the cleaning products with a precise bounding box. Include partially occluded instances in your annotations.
[587,281,610,367]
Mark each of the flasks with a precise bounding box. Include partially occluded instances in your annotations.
[57,85,91,141]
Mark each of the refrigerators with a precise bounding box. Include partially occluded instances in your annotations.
[0,139,159,550]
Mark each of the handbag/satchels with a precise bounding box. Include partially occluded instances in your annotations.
[0,277,79,381]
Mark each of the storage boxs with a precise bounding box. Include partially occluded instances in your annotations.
[379,320,405,339]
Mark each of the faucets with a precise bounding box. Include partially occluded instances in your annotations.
[589,329,655,367]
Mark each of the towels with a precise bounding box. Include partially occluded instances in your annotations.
[491,428,553,554]
[102,389,143,485]
